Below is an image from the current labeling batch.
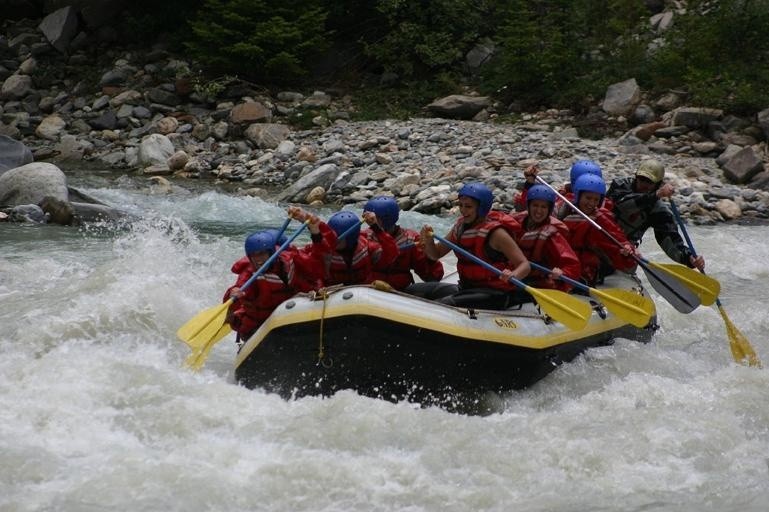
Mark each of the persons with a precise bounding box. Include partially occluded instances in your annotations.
[508,185,581,296]
[601,159,704,277]
[399,183,530,310]
[325,210,399,285]
[223,212,330,343]
[223,206,341,331]
[513,164,639,285]
[359,197,445,290]
[558,160,624,231]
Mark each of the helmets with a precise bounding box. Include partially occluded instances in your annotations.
[573,173,606,209]
[245,231,276,269]
[364,196,399,229]
[264,229,290,247]
[527,185,555,215]
[571,160,602,192]
[458,182,493,217]
[328,211,361,252]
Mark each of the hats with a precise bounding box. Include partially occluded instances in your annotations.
[636,160,664,184]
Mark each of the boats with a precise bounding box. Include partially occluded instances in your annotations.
[233,268,659,416]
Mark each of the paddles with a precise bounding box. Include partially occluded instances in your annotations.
[399,241,421,250]
[176,213,314,352]
[186,211,295,370]
[532,171,702,314]
[529,260,653,328]
[640,256,721,307]
[668,195,764,371]
[428,229,593,331]
[211,216,368,345]
